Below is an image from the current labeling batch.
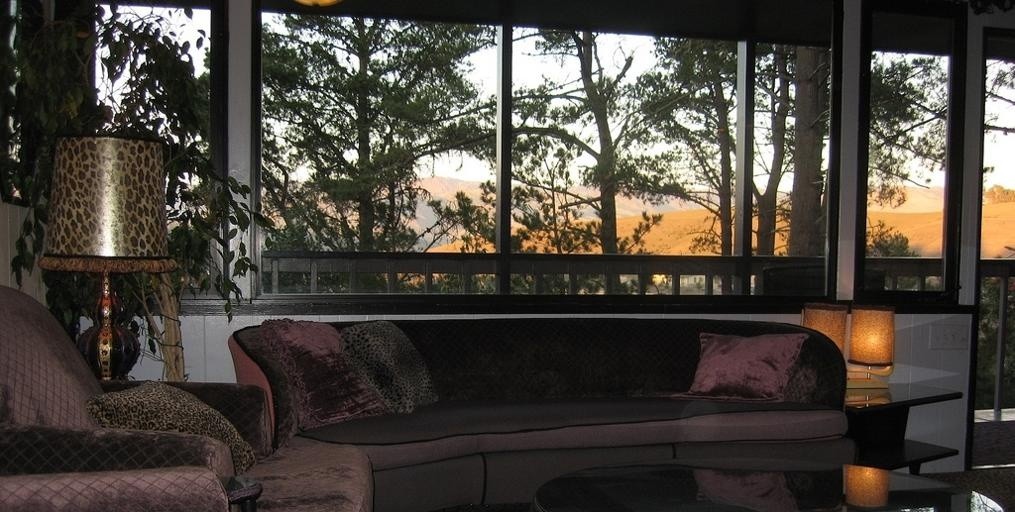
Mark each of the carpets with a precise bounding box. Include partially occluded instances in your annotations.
[972,413,1015,512]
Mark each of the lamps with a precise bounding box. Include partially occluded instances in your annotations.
[840,462,889,512]
[801,302,896,390]
[38,134,181,389]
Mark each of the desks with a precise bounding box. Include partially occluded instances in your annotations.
[888,472,972,511]
[535,459,903,511]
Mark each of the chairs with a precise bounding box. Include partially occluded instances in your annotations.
[0,466,262,512]
[0,282,376,510]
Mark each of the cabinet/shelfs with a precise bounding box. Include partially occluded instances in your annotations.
[841,384,963,476]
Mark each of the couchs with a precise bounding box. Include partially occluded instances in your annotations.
[227,315,856,510]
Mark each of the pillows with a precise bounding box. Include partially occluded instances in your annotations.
[251,353,301,448]
[260,318,388,441]
[688,329,812,400]
[783,366,818,398]
[85,380,257,474]
[692,467,797,511]
[339,317,442,416]
